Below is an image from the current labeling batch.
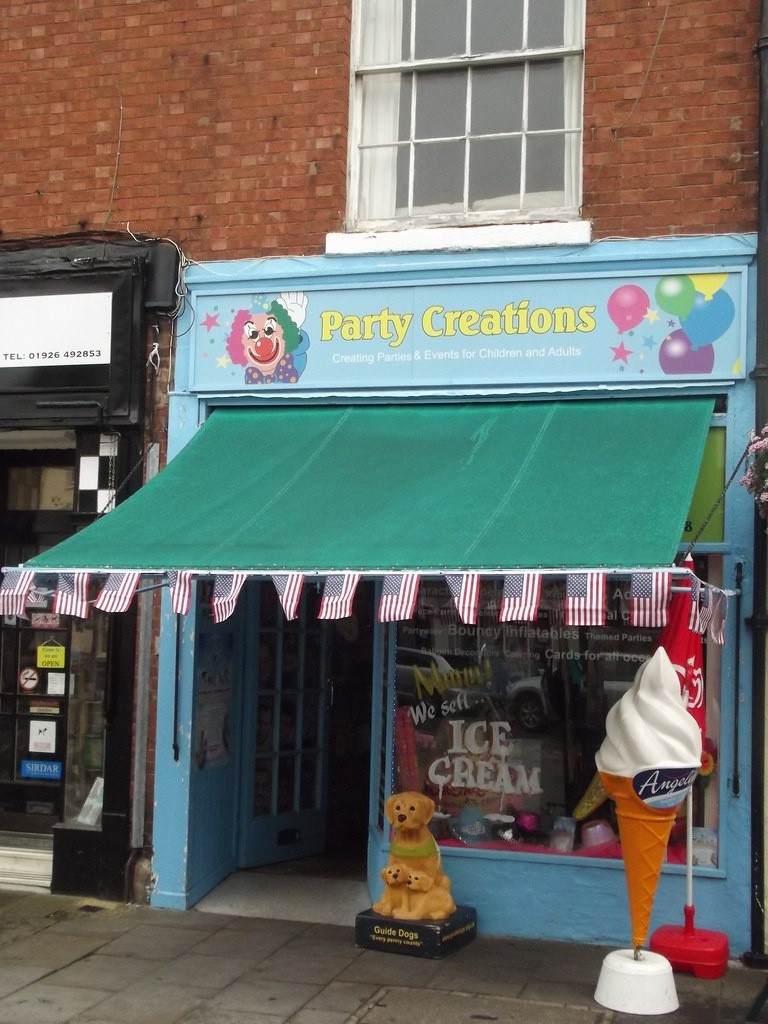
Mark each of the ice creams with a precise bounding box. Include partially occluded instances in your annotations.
[594,646,703,949]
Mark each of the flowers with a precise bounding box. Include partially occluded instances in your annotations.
[738,422,768,535]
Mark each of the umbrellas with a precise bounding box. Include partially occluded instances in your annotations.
[649,553,707,904]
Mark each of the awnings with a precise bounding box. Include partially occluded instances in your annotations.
[1,395,753,574]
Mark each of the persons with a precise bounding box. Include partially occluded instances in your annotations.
[535,635,609,755]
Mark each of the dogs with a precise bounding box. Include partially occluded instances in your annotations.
[371,790,457,921]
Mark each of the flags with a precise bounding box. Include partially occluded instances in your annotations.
[270,576,305,620]
[165,570,193,616]
[0,571,38,615]
[498,574,542,622]
[699,586,713,634]
[688,575,703,633]
[445,574,481,625]
[564,573,606,626]
[316,574,362,619]
[211,574,248,624]
[630,573,671,627]
[93,573,141,612]
[52,572,91,619]
[376,574,420,623]
